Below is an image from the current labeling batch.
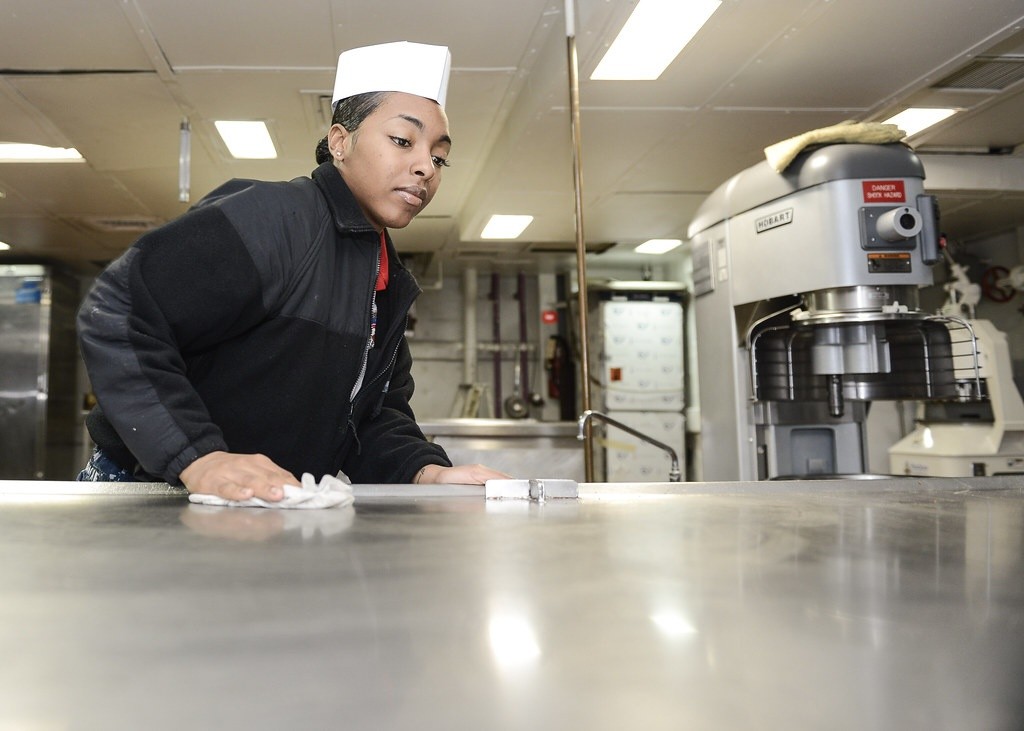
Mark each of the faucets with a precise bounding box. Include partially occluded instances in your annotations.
[575,408,683,481]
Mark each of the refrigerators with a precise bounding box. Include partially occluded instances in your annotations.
[576,301,687,482]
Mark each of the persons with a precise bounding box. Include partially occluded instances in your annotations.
[75,39,519,502]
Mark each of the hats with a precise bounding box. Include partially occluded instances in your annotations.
[331,41,451,112]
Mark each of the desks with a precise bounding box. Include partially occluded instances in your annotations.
[0,477,1024,731]
